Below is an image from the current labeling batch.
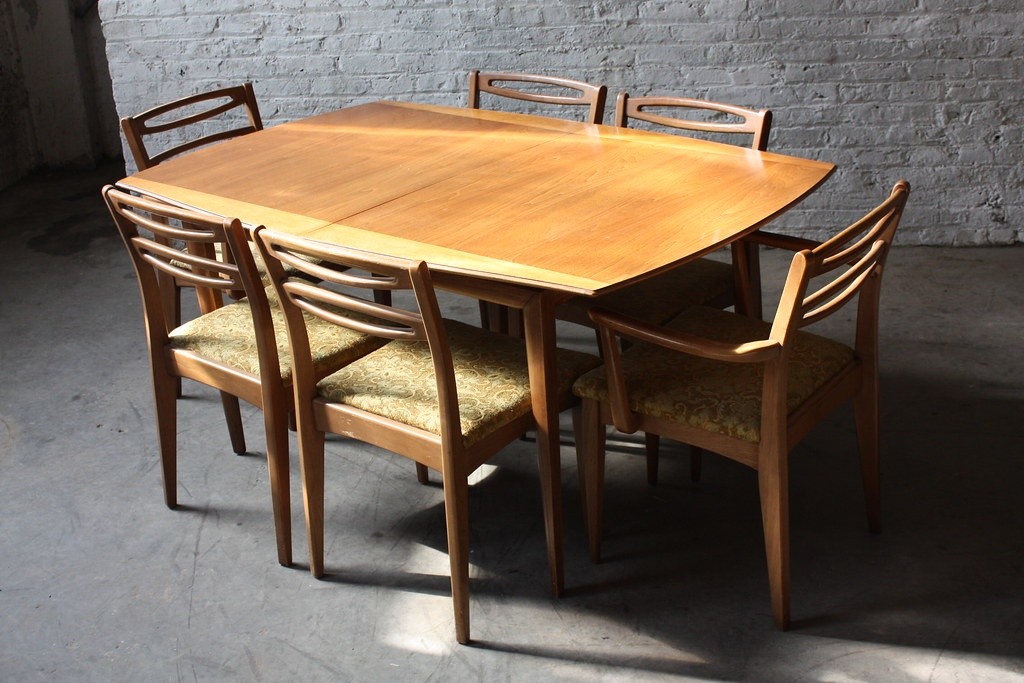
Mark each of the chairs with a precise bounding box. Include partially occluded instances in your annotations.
[566,178,909,633]
[248,222,603,644]
[512,93,772,489]
[464,68,606,341]
[121,83,389,399]
[102,186,430,567]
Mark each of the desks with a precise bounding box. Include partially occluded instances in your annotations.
[114,99,834,596]
